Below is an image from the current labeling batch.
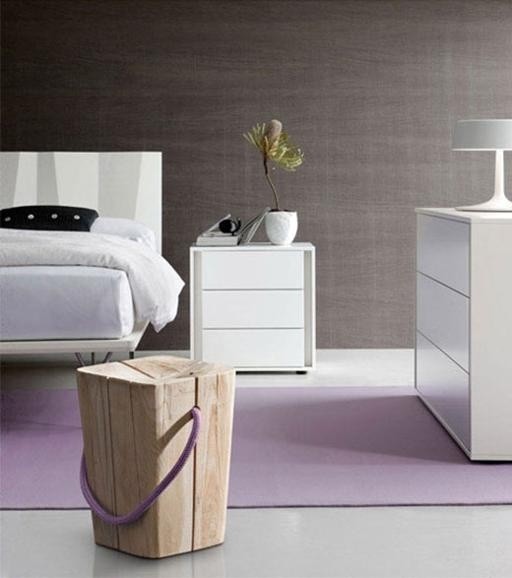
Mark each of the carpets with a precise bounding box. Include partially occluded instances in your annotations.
[0,387,512,509]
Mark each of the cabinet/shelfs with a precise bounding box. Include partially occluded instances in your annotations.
[414,208,512,461]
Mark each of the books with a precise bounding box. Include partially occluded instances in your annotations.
[234,207,271,246]
[201,214,234,236]
[195,234,242,247]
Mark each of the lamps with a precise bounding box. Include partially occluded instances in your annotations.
[451,119,512,211]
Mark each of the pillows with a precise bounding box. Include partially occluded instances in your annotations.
[0,205,98,231]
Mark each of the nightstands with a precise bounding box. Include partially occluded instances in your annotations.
[188,242,316,374]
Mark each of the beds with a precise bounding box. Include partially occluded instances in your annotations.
[1,150,163,367]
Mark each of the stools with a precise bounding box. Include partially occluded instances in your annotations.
[77,354,235,559]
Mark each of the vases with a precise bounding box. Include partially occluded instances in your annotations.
[265,209,299,246]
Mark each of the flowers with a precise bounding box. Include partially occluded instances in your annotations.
[244,120,305,210]
